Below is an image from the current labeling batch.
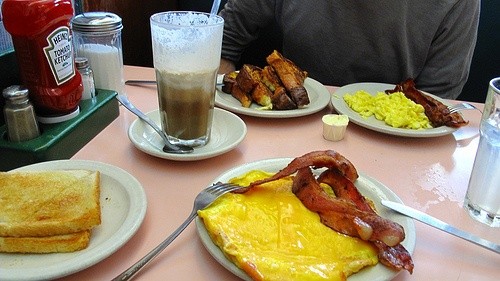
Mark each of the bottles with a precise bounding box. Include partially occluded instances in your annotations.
[0,1,128,143]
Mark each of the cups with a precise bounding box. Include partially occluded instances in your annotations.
[150,11,225,148]
[463,78,500,228]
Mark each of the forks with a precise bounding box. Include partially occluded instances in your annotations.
[111,183,244,281]
[447,102,483,115]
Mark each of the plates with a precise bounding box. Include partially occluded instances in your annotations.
[0,160,148,281]
[195,157,416,281]
[331,82,465,137]
[214,71,331,118]
[128,107,247,161]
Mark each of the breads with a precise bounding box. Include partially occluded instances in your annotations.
[222,50,310,110]
[0,169,102,254]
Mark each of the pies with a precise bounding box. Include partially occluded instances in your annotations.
[196,170,381,281]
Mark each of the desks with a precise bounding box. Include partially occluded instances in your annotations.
[53,65,500,280]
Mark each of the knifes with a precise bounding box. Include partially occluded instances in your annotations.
[125,80,224,87]
[380,201,500,255]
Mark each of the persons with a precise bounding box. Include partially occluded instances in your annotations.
[218,0,480,101]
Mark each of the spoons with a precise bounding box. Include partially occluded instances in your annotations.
[115,93,193,154]
